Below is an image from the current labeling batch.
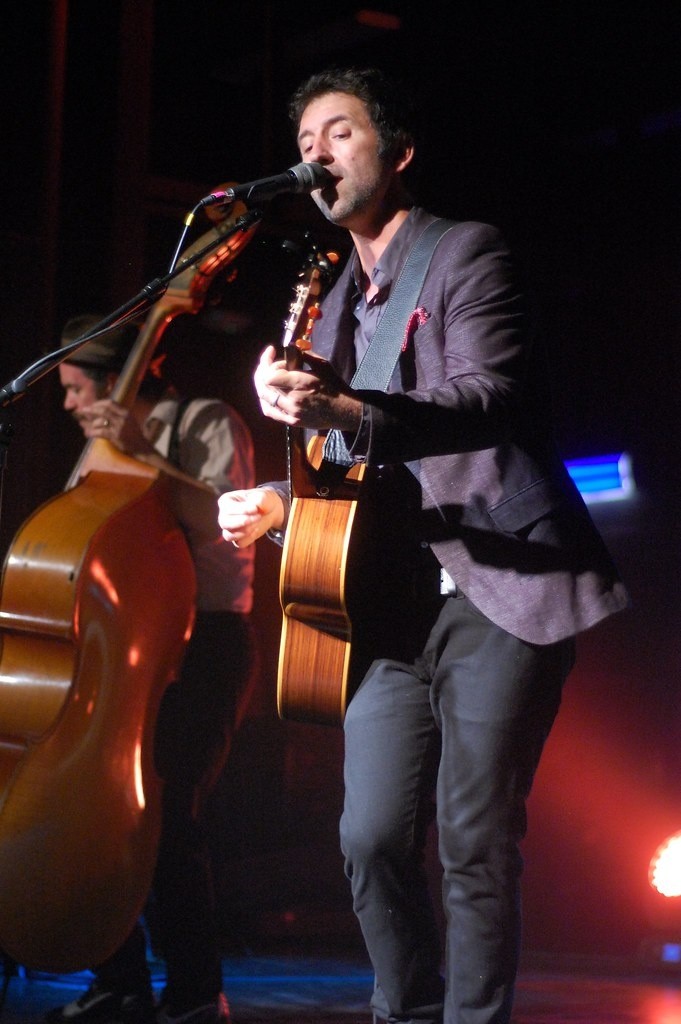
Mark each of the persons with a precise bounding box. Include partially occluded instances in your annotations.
[46,314,257,1024]
[218,68,629,1024]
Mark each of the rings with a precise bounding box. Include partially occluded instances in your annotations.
[104,420,109,427]
[268,393,281,407]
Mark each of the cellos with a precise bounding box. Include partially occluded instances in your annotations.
[0,181,266,977]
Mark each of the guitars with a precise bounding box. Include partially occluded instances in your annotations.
[277,243,396,728]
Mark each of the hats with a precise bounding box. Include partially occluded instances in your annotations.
[42,315,142,371]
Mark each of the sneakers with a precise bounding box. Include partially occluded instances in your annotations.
[145,985,230,1024]
[46,984,157,1024]
